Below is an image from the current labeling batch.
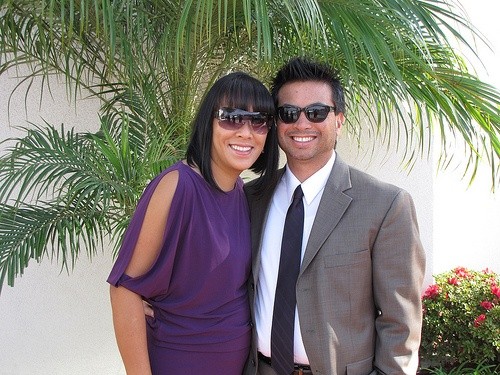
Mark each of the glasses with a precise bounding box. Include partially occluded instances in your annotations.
[278,104,337,123]
[214,106,274,135]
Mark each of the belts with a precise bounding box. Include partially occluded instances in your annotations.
[258,351,313,372]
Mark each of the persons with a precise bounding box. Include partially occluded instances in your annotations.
[241,56,426,375]
[106,73,280,375]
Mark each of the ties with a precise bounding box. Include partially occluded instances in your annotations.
[271,185,305,373]
